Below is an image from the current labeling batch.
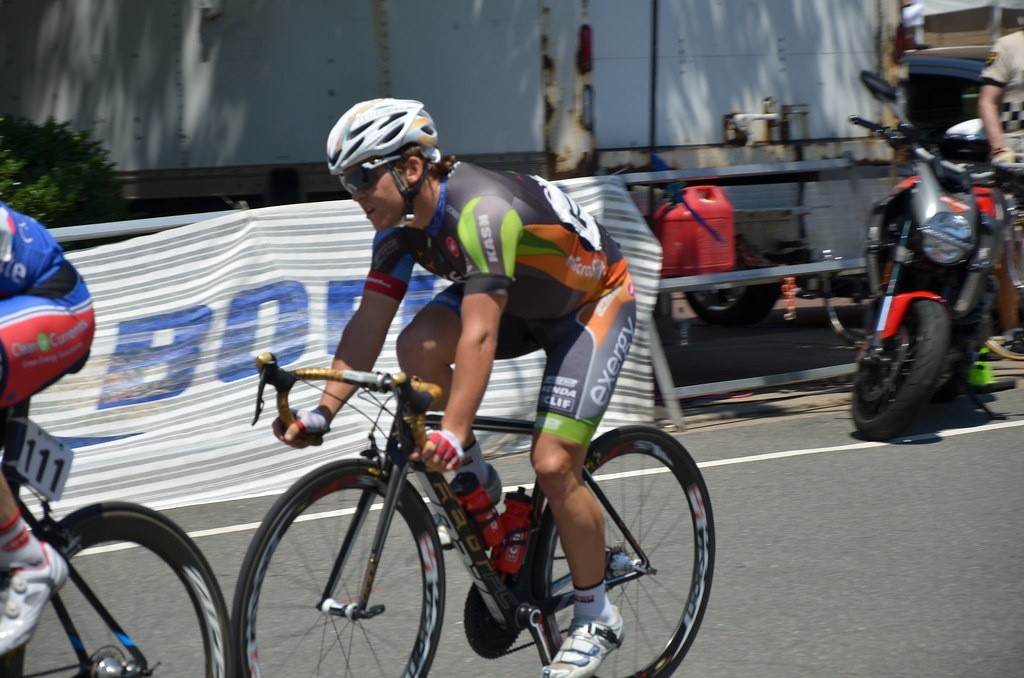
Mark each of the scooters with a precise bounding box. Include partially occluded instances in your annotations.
[846,63,1024,441]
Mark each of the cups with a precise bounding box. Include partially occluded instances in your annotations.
[783,104,809,142]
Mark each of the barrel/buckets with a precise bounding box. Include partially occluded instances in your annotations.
[653,185,735,277]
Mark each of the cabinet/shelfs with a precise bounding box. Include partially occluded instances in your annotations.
[621,158,913,432]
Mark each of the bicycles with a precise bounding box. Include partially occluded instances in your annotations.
[2,344,229,677]
[231,351,715,677]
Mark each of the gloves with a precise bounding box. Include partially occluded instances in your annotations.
[991,147,1015,165]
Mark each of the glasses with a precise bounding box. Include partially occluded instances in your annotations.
[339,155,401,193]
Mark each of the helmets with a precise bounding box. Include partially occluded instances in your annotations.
[327,97,441,176]
[943,118,991,162]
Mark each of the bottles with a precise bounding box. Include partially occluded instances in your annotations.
[489,487,534,574]
[450,471,505,547]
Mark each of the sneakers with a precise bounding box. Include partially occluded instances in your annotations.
[540,605,625,678]
[0,543,68,654]
[432,463,502,550]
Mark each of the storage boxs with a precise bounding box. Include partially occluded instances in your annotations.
[907,56,984,127]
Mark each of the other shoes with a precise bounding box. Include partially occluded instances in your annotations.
[1000,330,1024,354]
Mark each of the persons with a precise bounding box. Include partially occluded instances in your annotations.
[271,97,636,678]
[0,203,95,657]
[977,27,1024,354]
